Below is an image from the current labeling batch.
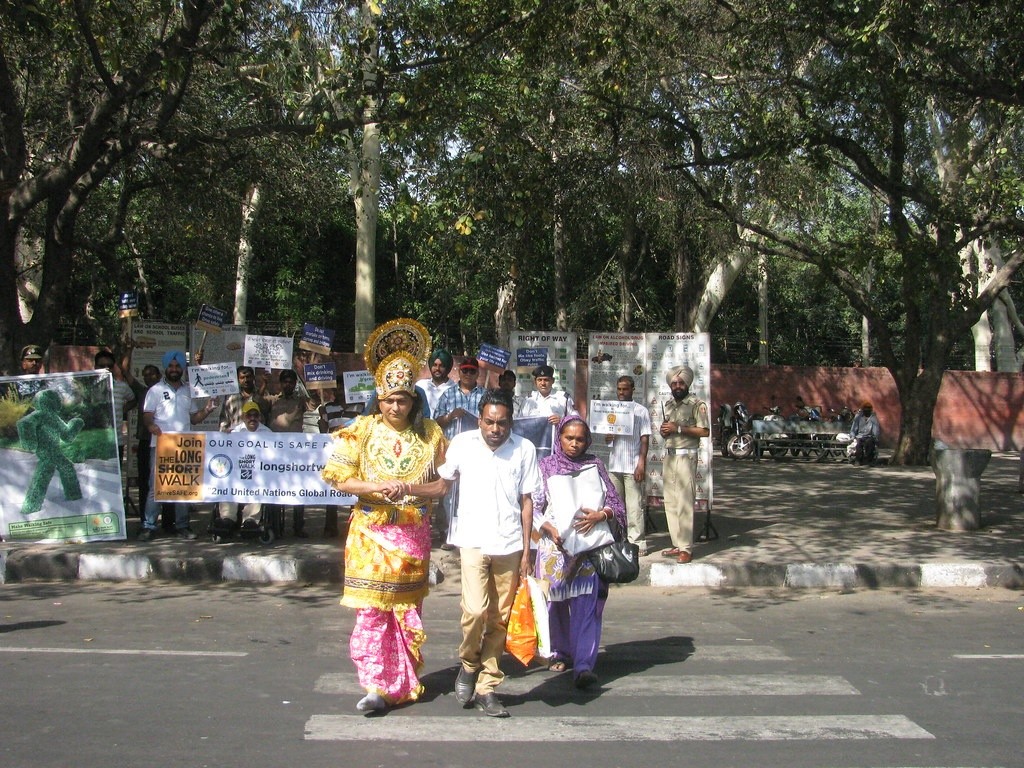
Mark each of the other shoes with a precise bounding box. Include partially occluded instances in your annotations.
[136,508,338,541]
[548,656,566,671]
[356,692,385,710]
[575,669,598,688]
[638,549,648,557]
[441,543,455,549]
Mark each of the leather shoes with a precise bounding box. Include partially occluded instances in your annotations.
[473,690,511,718]
[662,547,679,556]
[677,551,693,563]
[455,665,477,706]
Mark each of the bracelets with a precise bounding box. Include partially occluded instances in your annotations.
[446,414,450,423]
[407,483,412,496]
[601,509,611,521]
[678,426,681,434]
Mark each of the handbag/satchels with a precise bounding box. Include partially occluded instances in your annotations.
[526,574,553,659]
[503,572,538,668]
[585,526,640,583]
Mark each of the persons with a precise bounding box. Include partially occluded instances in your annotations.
[605,375,653,556]
[321,352,450,711]
[660,365,710,563]
[383,387,541,717]
[849,404,882,466]
[20,343,580,551]
[537,416,627,688]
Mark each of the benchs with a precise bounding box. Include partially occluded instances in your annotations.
[752,419,853,462]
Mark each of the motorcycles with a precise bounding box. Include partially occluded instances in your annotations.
[792,401,833,465]
[757,405,791,461]
[830,405,856,463]
[719,401,755,461]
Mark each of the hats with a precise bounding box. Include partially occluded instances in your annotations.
[242,402,261,418]
[460,357,479,369]
[163,351,186,370]
[365,318,432,400]
[531,365,554,378]
[20,345,44,362]
[429,348,453,374]
[666,366,694,388]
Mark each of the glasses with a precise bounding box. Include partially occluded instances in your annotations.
[247,410,259,417]
[460,368,476,375]
[97,363,114,368]
